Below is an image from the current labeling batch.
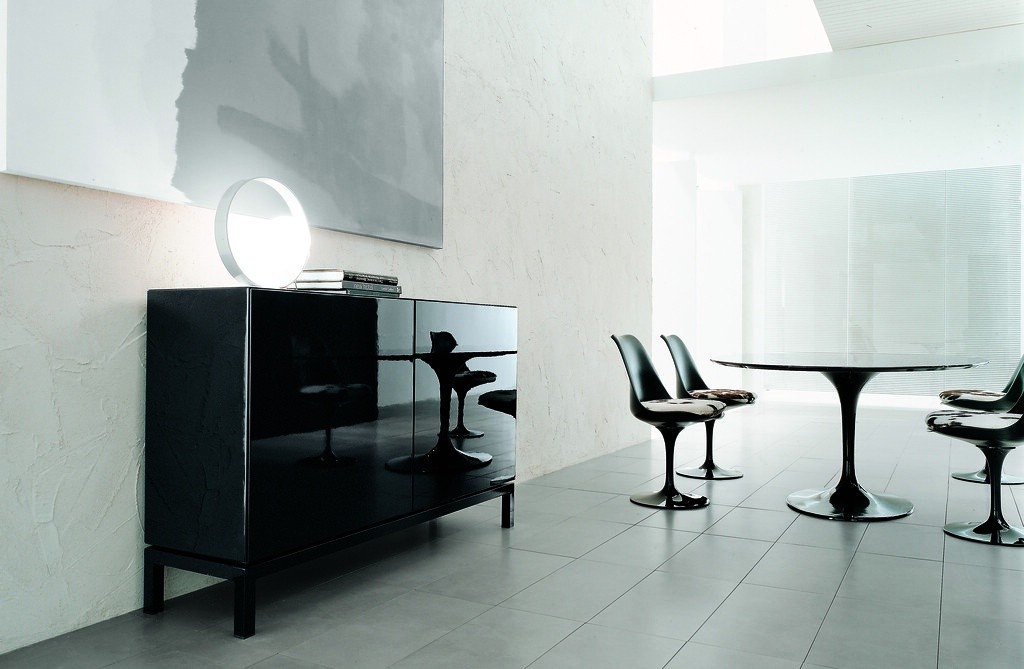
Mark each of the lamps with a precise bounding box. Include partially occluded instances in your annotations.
[213,175,311,291]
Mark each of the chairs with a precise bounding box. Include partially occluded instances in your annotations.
[924,351,1024,548]
[428,330,517,487]
[284,334,373,470]
[610,334,758,516]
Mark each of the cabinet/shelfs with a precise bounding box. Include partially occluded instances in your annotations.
[142,288,520,640]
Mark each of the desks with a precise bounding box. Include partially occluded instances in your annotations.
[708,357,991,525]
[377,351,517,475]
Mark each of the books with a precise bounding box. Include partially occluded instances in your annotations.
[293,281,402,298]
[295,268,398,285]
